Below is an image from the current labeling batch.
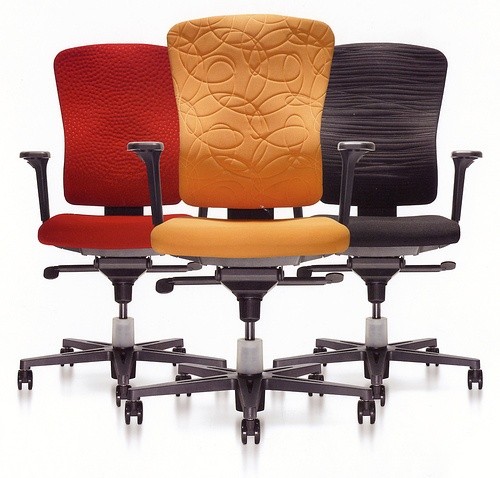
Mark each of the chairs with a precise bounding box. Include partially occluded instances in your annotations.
[16,42,195,403]
[123,12,382,447]
[273,42,484,408]
